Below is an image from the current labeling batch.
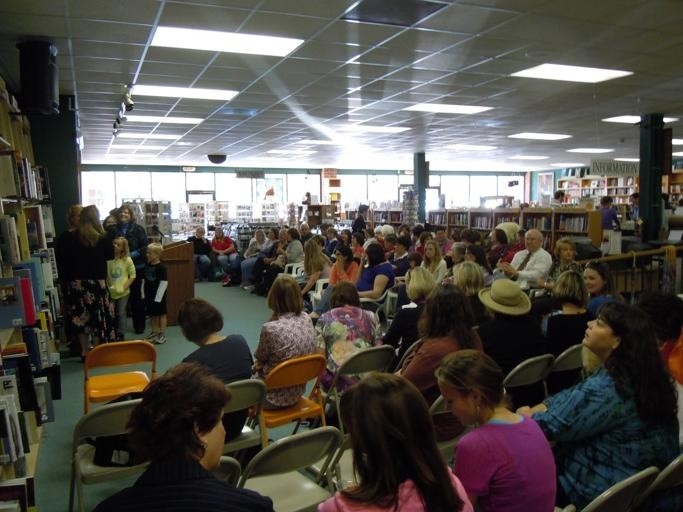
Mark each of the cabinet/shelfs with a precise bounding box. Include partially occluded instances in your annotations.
[373,211,403,230]
[557,169,683,216]
[120,197,172,245]
[1,91,62,512]
[345,211,372,230]
[307,205,336,228]
[429,208,601,256]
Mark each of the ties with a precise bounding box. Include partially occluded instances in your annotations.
[509,253,531,281]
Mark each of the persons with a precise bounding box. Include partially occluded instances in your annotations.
[55,177,683,512]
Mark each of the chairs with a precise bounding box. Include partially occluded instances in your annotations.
[282,262,335,308]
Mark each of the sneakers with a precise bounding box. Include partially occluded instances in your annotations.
[154,335,166,344]
[143,333,158,343]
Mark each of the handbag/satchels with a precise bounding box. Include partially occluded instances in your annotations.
[84,391,148,467]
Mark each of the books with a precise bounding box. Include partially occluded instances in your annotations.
[0,148,63,512]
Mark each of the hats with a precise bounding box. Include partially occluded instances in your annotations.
[478,278,532,316]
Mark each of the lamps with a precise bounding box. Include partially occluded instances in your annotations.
[112,92,134,136]
